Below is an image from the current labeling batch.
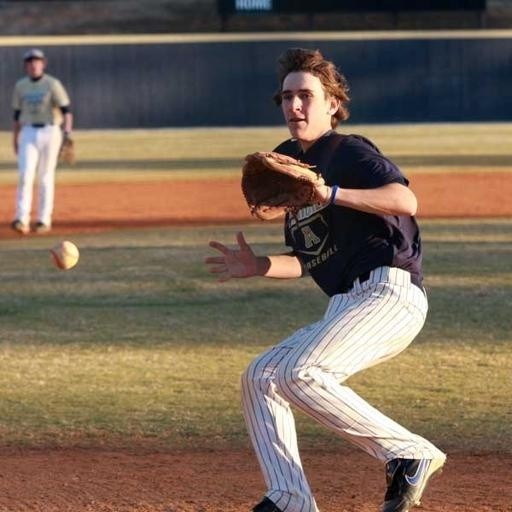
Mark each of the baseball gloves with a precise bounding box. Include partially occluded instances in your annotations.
[58,135,74,164]
[242,151,325,220]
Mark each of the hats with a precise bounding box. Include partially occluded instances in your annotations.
[23,48,44,60]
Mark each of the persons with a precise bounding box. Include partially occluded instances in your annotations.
[204,49,447,512]
[11,49,73,234]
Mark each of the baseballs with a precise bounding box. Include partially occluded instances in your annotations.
[50,242,80,270]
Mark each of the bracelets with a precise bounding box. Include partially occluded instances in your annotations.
[321,185,329,204]
[330,187,338,206]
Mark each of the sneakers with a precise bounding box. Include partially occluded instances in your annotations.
[382,458,445,512]
